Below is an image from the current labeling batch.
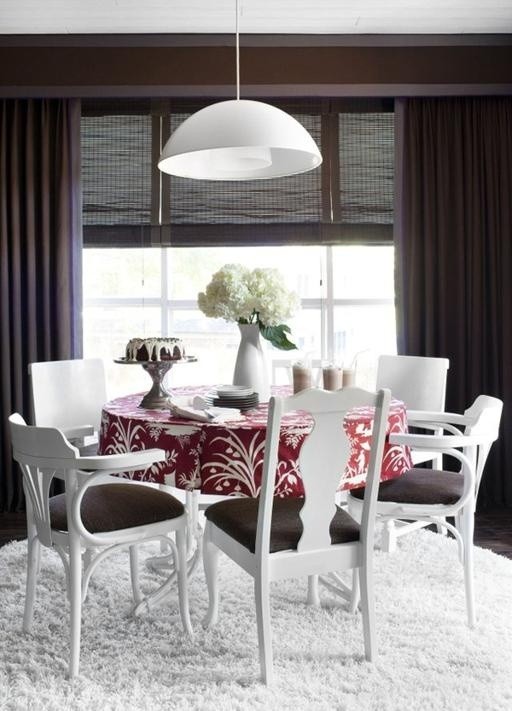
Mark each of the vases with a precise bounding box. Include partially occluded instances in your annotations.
[233,323,271,403]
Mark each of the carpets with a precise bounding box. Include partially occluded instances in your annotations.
[0,527,510,706]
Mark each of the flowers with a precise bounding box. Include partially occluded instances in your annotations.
[198,264,297,351]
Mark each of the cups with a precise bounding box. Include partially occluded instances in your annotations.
[322,365,339,392]
[292,364,312,394]
[342,368,356,388]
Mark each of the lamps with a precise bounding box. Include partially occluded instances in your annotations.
[157,1,324,183]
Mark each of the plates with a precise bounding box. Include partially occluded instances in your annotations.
[204,383,260,411]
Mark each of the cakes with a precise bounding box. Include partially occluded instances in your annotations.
[125,337,186,362]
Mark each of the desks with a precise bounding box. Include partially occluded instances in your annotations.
[98,382,412,625]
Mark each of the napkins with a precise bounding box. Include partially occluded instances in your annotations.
[164,396,246,422]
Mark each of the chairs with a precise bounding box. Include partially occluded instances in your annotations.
[349,395,502,629]
[8,412,194,678]
[203,387,392,686]
[29,358,107,456]
[375,355,449,547]
